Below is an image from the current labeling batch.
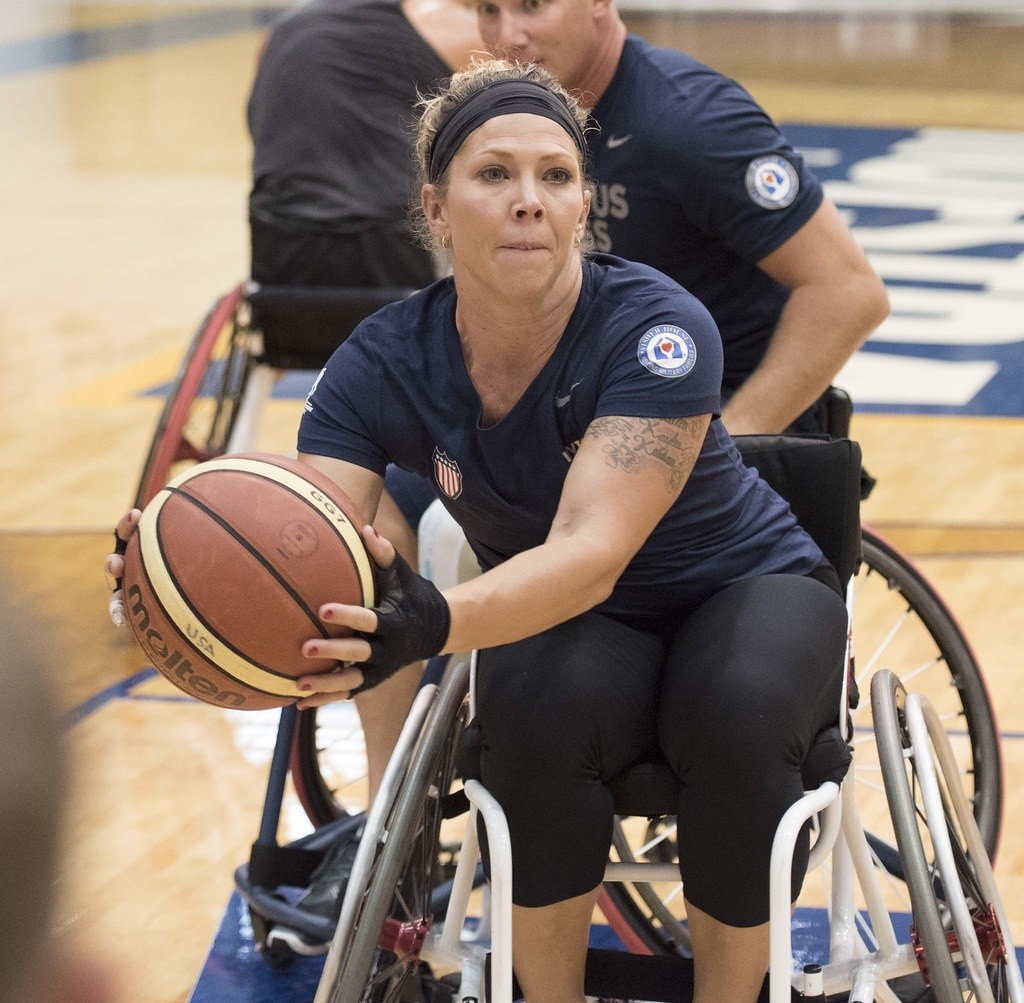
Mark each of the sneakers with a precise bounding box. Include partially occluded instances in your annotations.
[264,831,389,956]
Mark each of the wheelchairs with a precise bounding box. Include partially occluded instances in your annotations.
[228,383,1024,1003]
[122,277,444,523]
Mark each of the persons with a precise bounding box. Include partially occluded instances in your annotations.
[244,0,494,368]
[102,60,849,1003]
[267,0,893,959]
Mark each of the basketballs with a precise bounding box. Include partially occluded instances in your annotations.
[120,449,382,712]
